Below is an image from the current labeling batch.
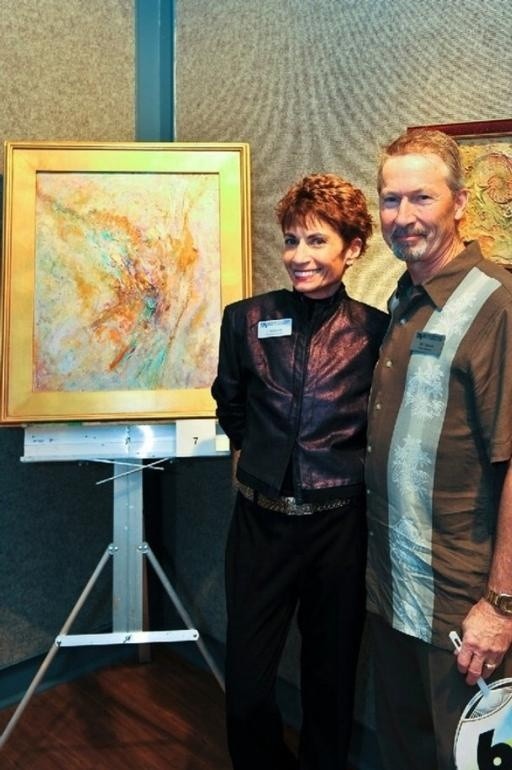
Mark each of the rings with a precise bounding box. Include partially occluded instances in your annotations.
[485,662,499,669]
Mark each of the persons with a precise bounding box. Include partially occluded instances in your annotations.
[361,128,510,770]
[210,172,391,770]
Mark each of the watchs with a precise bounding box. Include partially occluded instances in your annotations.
[482,586,511,616]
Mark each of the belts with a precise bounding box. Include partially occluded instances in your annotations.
[233,478,357,518]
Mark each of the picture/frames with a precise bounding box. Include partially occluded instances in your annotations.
[405,118,512,272]
[0,139,253,424]
